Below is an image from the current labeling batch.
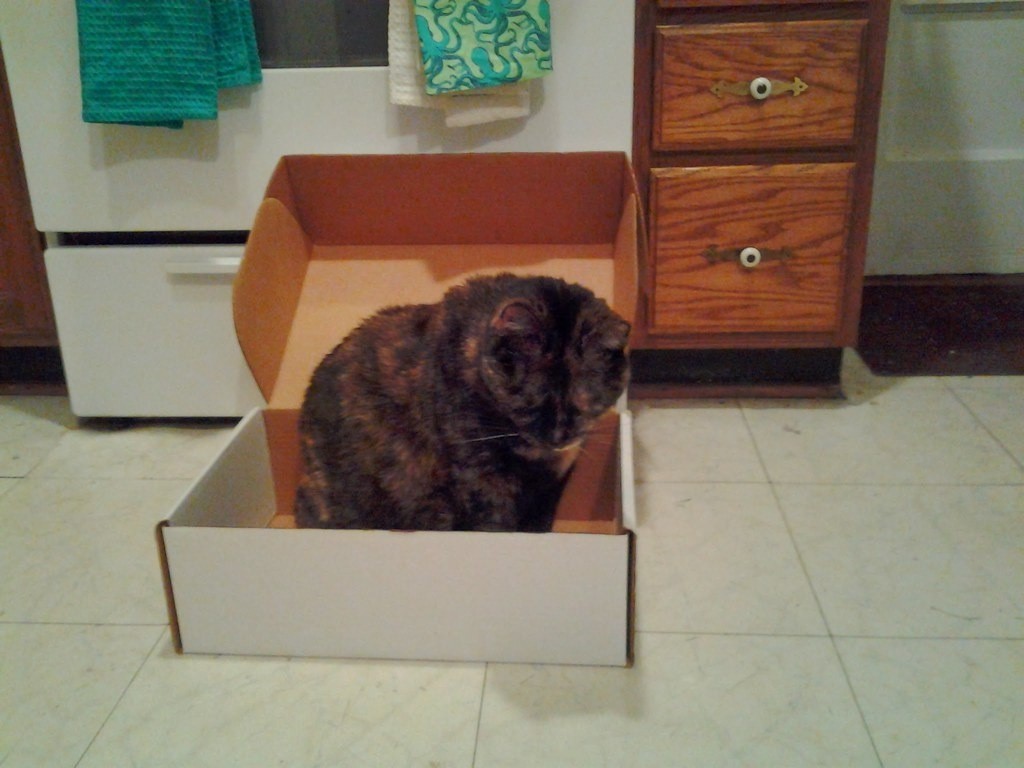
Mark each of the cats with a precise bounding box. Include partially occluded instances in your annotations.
[292,273,632,533]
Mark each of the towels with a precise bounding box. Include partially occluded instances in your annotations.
[70,0,267,132]
[383,0,555,129]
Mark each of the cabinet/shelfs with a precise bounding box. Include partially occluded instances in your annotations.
[0,0,891,426]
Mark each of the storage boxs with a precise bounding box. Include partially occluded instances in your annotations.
[156,151,647,670]
